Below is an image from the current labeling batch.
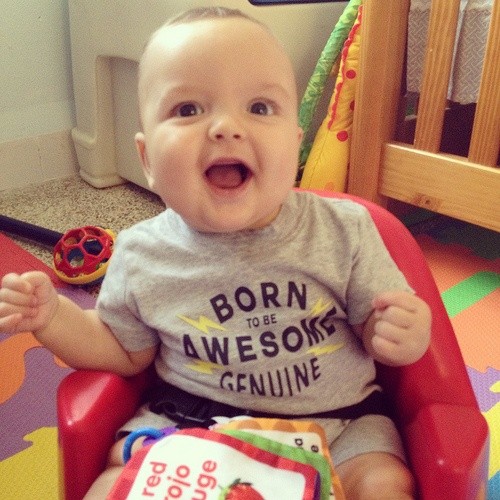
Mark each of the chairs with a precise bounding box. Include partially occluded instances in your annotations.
[55,188,490,500]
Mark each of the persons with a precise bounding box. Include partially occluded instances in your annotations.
[0,5,431,500]
